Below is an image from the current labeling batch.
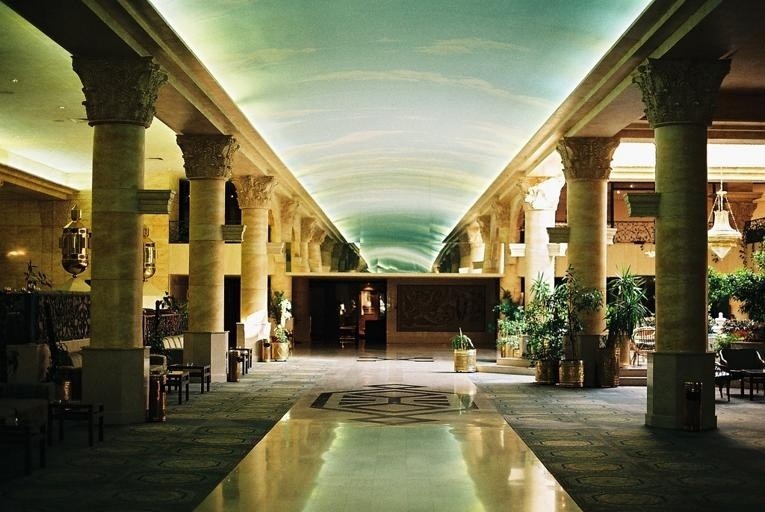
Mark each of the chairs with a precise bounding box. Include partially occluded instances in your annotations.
[629,323,765,403]
[1,328,252,480]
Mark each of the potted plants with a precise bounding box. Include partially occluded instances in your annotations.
[707,234,765,350]
[448,263,649,391]
[269,289,292,362]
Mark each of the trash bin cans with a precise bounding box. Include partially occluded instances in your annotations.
[262,338,271,362]
[227,350,240,382]
[679,381,703,437]
[147,374,167,422]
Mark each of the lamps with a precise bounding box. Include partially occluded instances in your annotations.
[707,143,743,262]
[57,203,157,287]
